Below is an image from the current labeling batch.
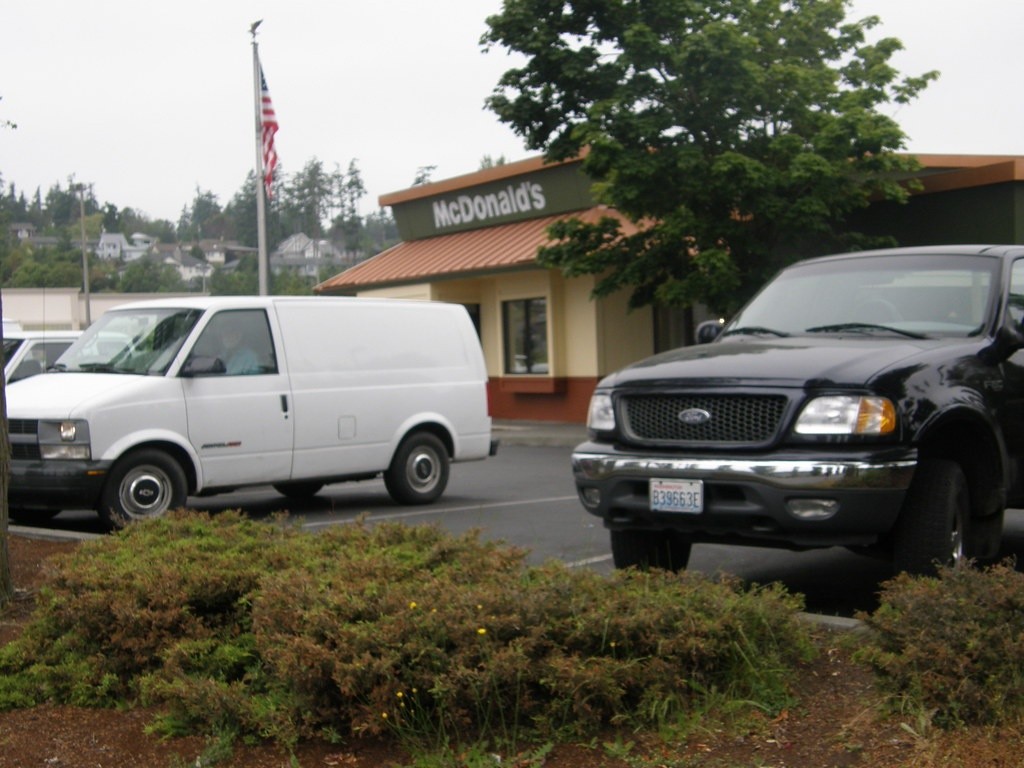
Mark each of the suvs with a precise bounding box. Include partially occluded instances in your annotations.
[571,243,1024,576]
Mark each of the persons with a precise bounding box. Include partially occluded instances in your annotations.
[214,320,258,374]
[31,343,53,372]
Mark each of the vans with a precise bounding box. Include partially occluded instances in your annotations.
[5,296,491,530]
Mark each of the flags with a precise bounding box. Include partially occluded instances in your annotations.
[259,65,280,198]
